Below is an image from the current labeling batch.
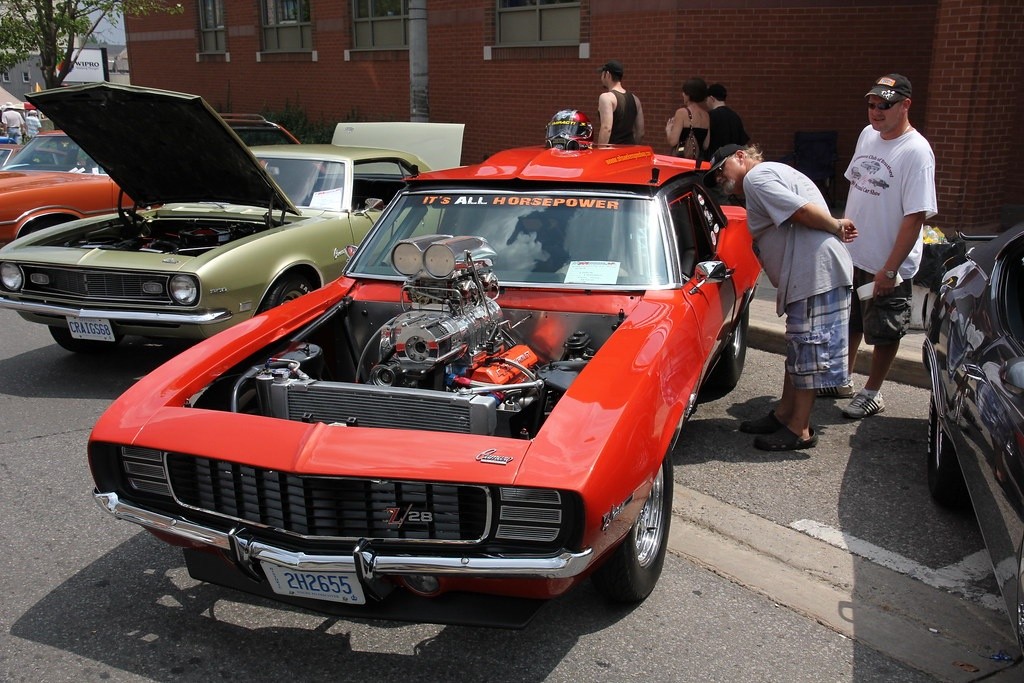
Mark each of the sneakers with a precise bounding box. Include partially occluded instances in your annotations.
[815,381,855,398]
[842,393,886,418]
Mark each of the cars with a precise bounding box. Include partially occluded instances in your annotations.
[88,109,758,603]
[0,82,465,352]
[0,145,87,172]
[0,131,134,248]
[920,225,1023,672]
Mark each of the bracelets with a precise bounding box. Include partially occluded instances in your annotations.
[834,218,842,236]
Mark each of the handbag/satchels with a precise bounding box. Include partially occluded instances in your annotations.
[671,107,699,160]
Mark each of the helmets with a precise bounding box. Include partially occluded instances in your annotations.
[545,107,594,151]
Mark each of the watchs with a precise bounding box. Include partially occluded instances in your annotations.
[881,268,897,279]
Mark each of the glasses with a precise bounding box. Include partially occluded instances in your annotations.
[713,155,735,177]
[868,99,902,110]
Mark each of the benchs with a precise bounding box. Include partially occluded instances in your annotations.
[308,176,407,208]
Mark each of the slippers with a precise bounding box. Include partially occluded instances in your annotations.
[740,409,787,434]
[754,425,819,452]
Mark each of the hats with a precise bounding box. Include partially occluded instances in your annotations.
[4,102,16,108]
[705,83,727,100]
[703,143,749,188]
[864,74,912,102]
[595,60,624,76]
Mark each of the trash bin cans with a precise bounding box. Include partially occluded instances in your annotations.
[913,238,966,288]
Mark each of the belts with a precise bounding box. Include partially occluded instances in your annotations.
[7,127,19,128]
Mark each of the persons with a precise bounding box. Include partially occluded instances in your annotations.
[815,74,938,419]
[706,84,750,161]
[702,142,858,451]
[666,77,710,160]
[595,61,645,147]
[25,110,42,138]
[0,101,25,145]
